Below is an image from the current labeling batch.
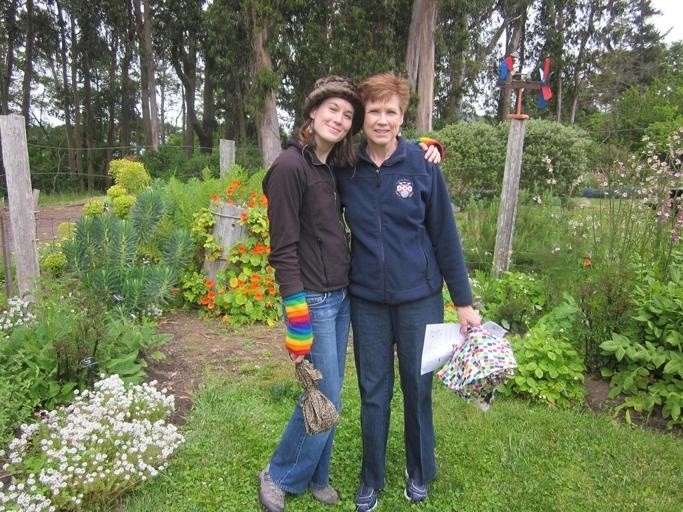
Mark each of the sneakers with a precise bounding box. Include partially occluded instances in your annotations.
[356,482,377,511]
[258,464,286,512]
[404,469,428,502]
[309,482,338,504]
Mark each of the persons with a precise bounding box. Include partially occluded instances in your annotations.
[330,73,481,512]
[258,74,445,512]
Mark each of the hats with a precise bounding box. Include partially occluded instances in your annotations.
[302,75,364,136]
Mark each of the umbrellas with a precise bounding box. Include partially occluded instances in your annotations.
[288,350,339,435]
[436,325,518,413]
